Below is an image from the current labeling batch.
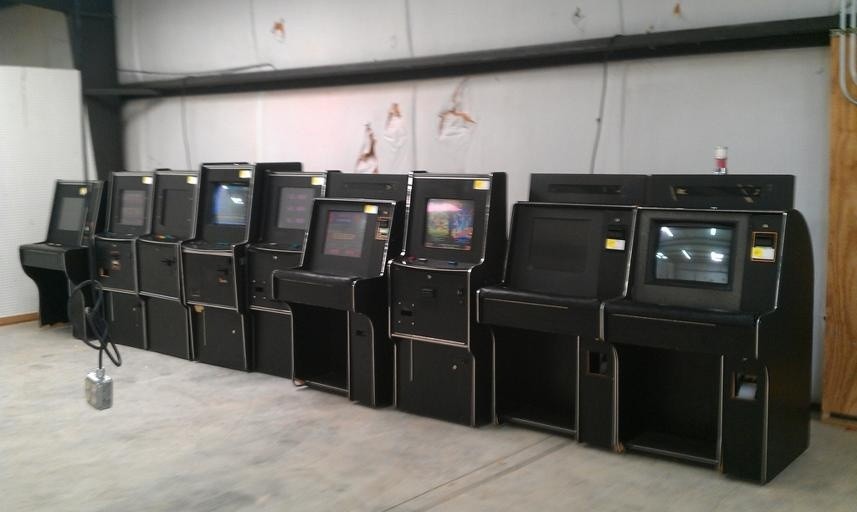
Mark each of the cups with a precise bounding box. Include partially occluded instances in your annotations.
[713,145,728,175]
